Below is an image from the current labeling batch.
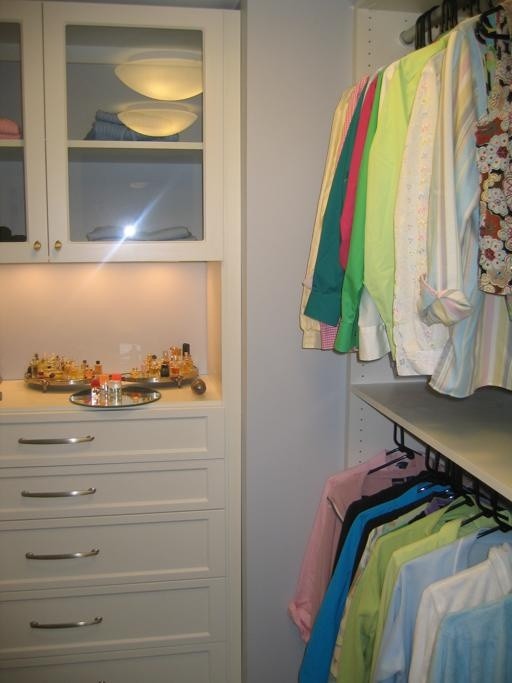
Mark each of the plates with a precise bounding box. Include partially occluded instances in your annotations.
[70,385,161,407]
[111,367,200,387]
[25,366,106,392]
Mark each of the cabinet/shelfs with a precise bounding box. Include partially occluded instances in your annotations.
[2,406,244,682]
[1,0,241,260]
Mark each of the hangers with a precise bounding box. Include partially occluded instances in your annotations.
[414,0,510,49]
[366,420,511,541]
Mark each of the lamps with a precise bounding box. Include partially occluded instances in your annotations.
[113,47,203,138]
